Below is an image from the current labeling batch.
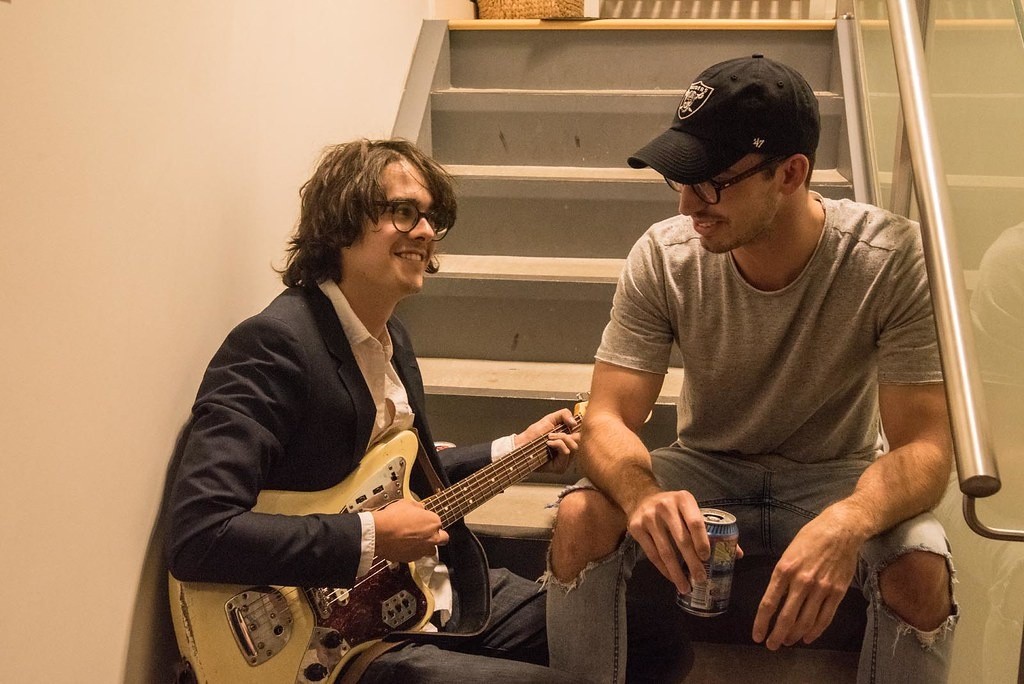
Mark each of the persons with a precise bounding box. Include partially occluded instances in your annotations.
[170,137,679,684]
[535,55,959,684]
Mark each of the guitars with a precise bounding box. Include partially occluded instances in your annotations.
[162,392,657,684]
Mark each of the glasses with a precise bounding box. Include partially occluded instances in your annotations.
[663,153,815,205]
[372,200,450,242]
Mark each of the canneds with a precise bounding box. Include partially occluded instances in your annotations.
[676,508,739,618]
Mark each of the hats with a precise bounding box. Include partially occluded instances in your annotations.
[627,54,821,184]
